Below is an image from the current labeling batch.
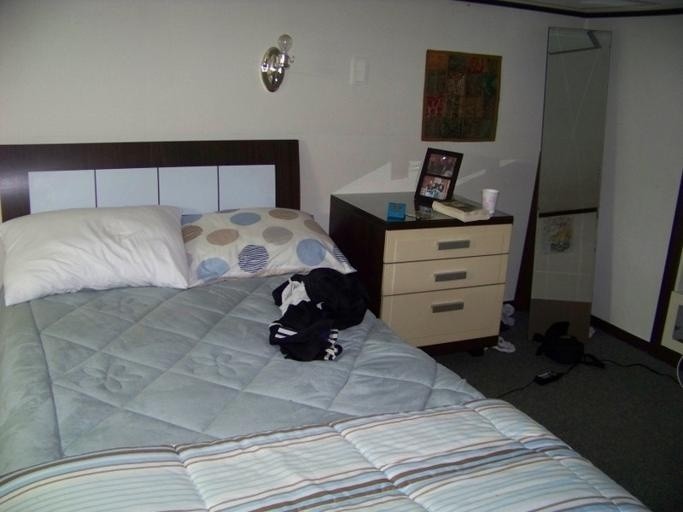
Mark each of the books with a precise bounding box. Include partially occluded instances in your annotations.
[431,200,489,224]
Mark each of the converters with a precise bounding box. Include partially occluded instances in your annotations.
[534,369,560,385]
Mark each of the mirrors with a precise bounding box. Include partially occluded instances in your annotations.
[523,27,609,351]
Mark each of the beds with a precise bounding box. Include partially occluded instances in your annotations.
[0,140,656,512]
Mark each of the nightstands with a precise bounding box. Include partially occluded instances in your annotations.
[327,192,513,357]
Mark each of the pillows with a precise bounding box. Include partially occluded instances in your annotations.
[181,206,357,291]
[1,206,189,309]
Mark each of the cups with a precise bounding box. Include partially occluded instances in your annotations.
[480,188,501,215]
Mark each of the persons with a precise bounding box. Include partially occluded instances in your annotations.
[425,156,455,193]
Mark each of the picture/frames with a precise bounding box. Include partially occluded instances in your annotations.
[414,149,463,207]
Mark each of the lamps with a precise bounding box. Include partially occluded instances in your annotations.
[262,33,291,92]
